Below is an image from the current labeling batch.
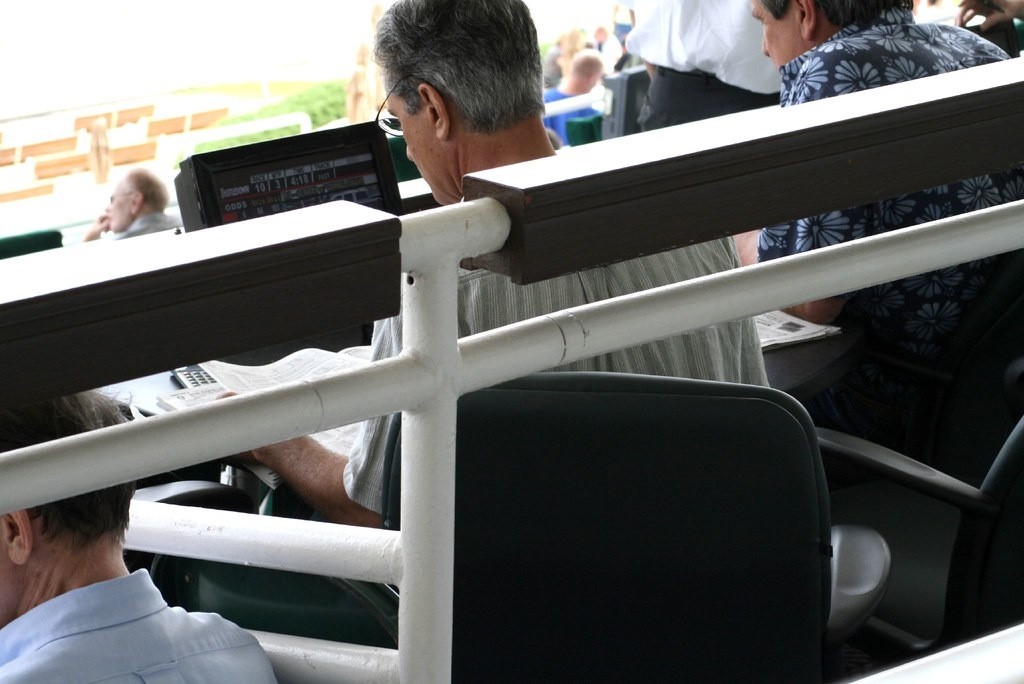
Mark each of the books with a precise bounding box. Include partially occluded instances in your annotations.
[155,342,379,489]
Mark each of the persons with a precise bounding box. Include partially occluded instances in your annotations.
[0,385,288,684]
[624,0,792,137]
[957,1,1024,34]
[85,160,182,248]
[530,2,648,159]
[185,0,774,649]
[728,1,1024,453]
[343,2,395,127]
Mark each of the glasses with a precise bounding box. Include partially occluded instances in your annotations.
[374,74,444,137]
[110,191,134,203]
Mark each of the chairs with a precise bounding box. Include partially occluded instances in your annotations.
[813,245,1024,667]
[148,371,834,684]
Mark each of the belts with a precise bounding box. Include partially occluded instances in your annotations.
[659,66,715,78]
